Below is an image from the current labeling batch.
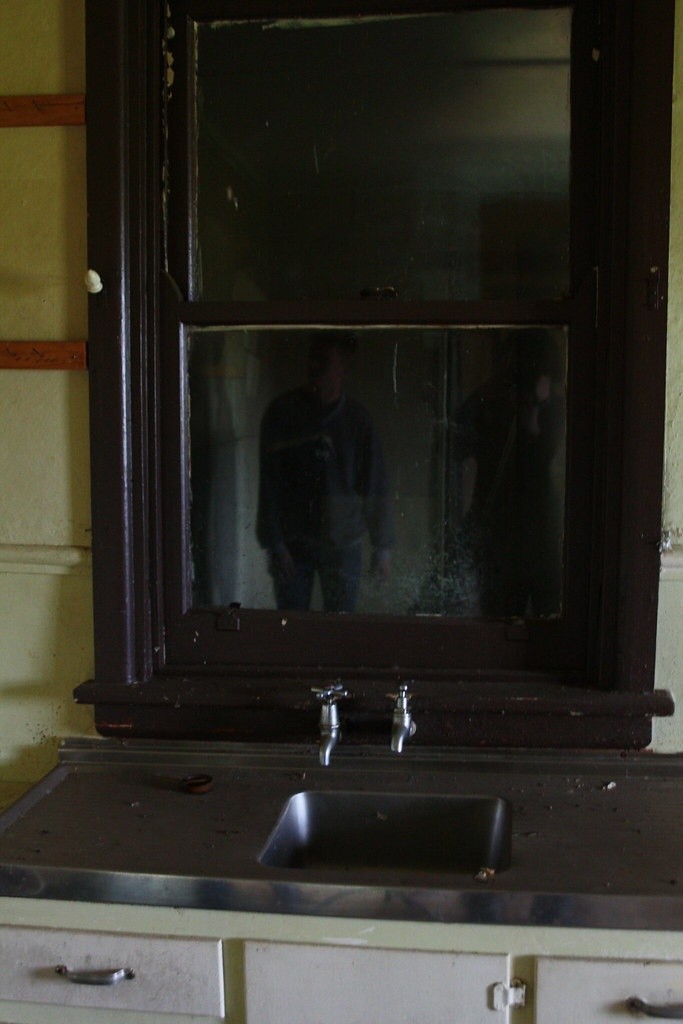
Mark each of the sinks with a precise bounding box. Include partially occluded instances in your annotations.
[256,788,516,880]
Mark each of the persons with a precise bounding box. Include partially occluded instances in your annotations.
[255,332,393,613]
[452,327,560,617]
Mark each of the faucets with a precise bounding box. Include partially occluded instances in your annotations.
[384,683,420,754]
[310,682,351,767]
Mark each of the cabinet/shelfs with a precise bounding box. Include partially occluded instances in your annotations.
[0,895,683,1024]
